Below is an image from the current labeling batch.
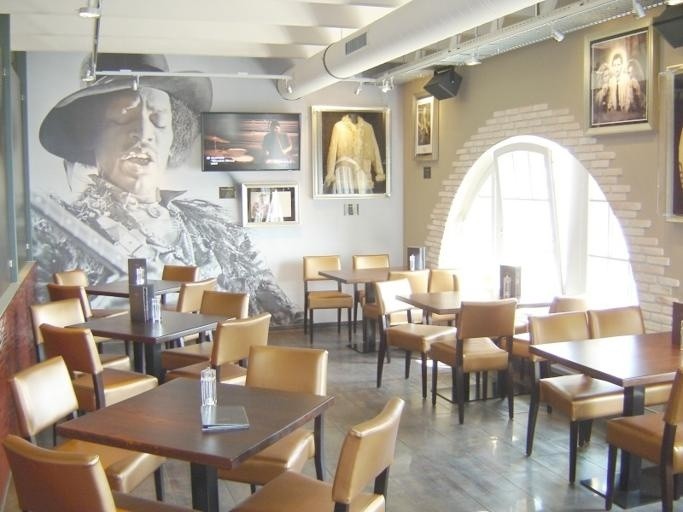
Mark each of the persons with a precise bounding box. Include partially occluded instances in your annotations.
[26,53,312,327]
[324,112,385,195]
[259,120,293,161]
[416,103,430,145]
[594,54,645,113]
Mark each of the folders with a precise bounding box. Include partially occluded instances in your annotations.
[201,405,249,430]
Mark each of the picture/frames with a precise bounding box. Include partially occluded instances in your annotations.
[583,16,683,222]
[311,106,391,200]
[242,183,300,228]
[412,91,438,162]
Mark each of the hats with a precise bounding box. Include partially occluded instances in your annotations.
[38,52,213,165]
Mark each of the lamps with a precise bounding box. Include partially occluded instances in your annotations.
[551,29,565,42]
[79,0,101,18]
[632,0,646,19]
[96,70,376,95]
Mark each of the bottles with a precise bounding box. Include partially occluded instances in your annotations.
[151,298,161,321]
[200,368,217,407]
[409,254,415,271]
[503,277,510,299]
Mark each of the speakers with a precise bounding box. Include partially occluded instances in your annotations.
[651,3,683,49]
[423,71,462,99]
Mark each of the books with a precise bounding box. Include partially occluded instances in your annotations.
[498,262,522,300]
[672,300,683,347]
[129,284,156,325]
[407,245,426,271]
[199,403,250,433]
[128,257,147,285]
